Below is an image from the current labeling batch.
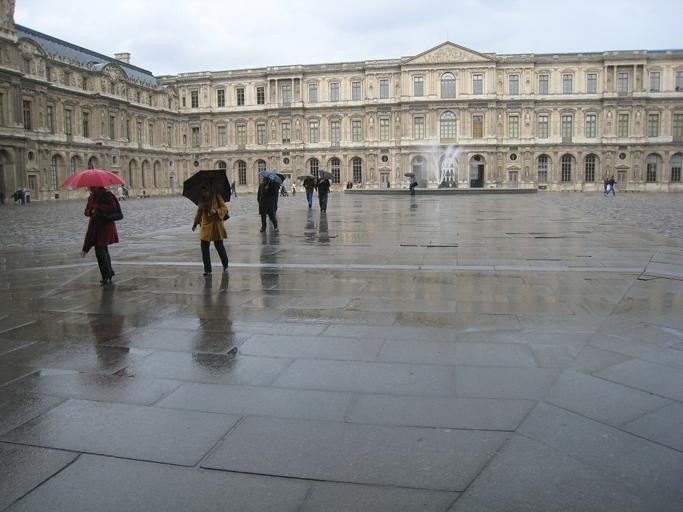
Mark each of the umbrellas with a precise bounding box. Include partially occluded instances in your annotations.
[260,171,282,185]
[318,169,333,180]
[59,168,124,188]
[182,171,232,216]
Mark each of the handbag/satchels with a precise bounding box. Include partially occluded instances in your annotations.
[215,192,230,221]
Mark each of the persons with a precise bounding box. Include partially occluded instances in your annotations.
[304,176,317,207]
[292,181,296,195]
[346,182,352,189]
[281,186,289,197]
[315,178,330,213]
[232,181,237,198]
[409,174,416,195]
[607,175,617,197]
[604,177,608,194]
[256,176,279,232]
[85,184,123,283]
[192,183,229,276]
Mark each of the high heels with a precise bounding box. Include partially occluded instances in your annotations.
[100,276,112,283]
